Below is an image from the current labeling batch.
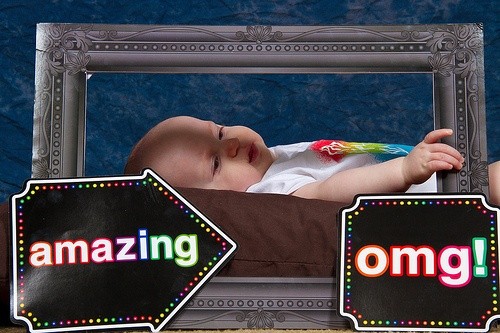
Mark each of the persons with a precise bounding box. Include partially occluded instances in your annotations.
[124,116,500,212]
